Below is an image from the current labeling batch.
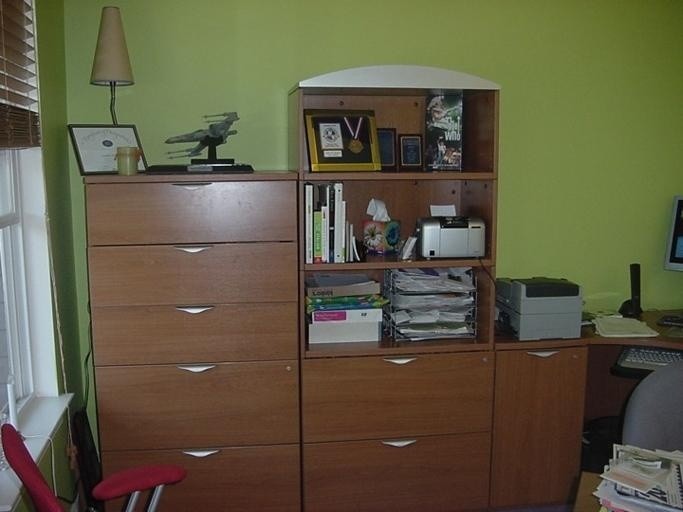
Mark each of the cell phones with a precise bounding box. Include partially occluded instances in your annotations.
[657,316,683,327]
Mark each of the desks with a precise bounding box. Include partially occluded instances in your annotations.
[496,299,683,509]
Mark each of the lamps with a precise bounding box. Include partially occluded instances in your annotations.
[88,6,134,125]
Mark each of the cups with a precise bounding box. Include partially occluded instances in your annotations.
[113,147,143,176]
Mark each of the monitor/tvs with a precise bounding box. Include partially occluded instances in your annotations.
[664,196,683,273]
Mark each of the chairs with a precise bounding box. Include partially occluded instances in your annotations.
[619,358,683,453]
[0,422,187,511]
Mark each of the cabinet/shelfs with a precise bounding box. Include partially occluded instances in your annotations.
[286,67,501,356]
[301,350,493,512]
[80,174,300,511]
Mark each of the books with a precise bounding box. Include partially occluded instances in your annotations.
[304,267,478,344]
[304,181,362,266]
[591,442,682,512]
[578,307,660,339]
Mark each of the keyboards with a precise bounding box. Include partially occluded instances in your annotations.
[617,347,683,370]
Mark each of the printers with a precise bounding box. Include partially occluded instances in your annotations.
[496,277,582,342]
[416,205,486,260]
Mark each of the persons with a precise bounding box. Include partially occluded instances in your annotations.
[425,95,462,169]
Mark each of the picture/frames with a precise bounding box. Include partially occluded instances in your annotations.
[65,123,146,176]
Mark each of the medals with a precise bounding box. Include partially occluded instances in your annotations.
[344,135,366,156]
